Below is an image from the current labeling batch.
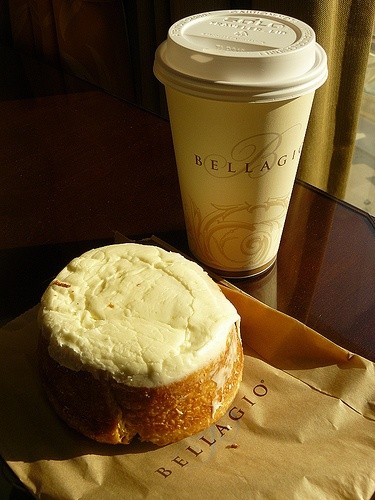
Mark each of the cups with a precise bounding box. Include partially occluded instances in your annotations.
[152,9,329,277]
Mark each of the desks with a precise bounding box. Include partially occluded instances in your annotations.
[0,47,375,500]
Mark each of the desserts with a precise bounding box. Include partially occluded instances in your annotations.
[37,242,244,445]
